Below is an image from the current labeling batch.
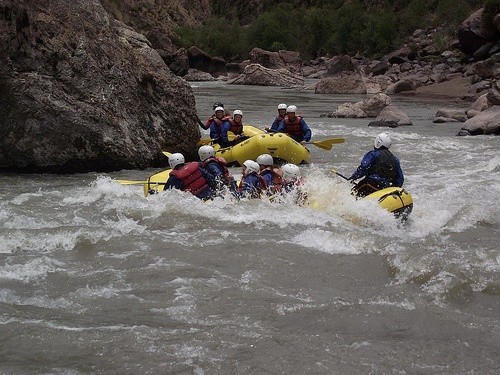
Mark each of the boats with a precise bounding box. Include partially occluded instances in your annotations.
[305,180,414,231]
[197,124,311,170]
[144,168,319,214]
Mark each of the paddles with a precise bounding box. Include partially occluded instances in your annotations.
[161,151,173,158]
[112,180,165,186]
[298,138,346,143]
[268,128,333,150]
[331,169,359,185]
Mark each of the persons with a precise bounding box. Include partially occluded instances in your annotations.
[232,160,261,199]
[163,152,213,200]
[277,105,311,145]
[266,103,288,132]
[256,154,275,191]
[347,133,404,196]
[221,109,249,147]
[197,145,232,191]
[268,163,306,206]
[196,101,228,144]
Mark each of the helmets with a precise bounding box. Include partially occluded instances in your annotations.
[242,159,260,176]
[212,102,224,110]
[256,153,273,166]
[198,145,215,162]
[286,105,298,115]
[168,153,185,169]
[374,132,392,150]
[277,103,288,110]
[280,163,300,179]
[214,106,225,112]
[232,109,243,120]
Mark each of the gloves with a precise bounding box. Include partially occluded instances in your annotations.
[148,189,158,196]
[196,114,200,122]
[346,176,357,184]
